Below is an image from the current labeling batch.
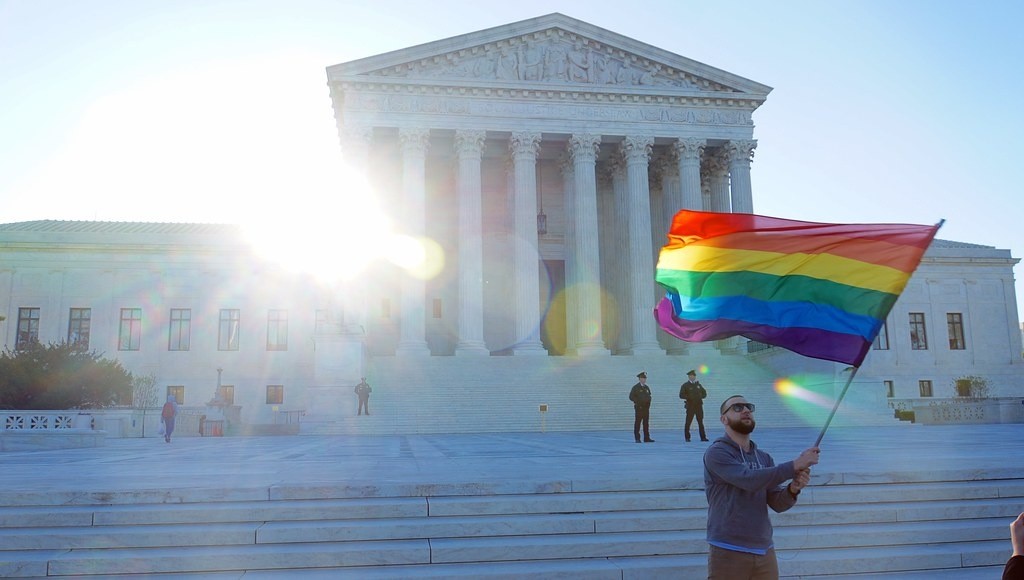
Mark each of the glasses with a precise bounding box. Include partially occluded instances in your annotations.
[722,403,755,415]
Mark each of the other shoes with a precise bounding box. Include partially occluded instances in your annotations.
[701,439,709,441]
[644,439,655,442]
[164,434,171,443]
[635,440,642,443]
[686,439,691,442]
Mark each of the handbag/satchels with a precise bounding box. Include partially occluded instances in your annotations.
[158,423,164,435]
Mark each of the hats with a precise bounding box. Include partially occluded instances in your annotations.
[361,377,367,380]
[686,370,695,375]
[636,372,647,378]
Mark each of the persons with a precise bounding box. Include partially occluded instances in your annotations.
[1002,512,1024,580]
[703,395,822,580]
[629,371,655,443]
[354,377,372,416]
[160,394,179,443]
[678,370,707,443]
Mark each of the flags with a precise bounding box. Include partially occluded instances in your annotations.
[654,209,939,371]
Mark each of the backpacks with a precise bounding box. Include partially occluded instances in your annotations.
[162,402,174,419]
